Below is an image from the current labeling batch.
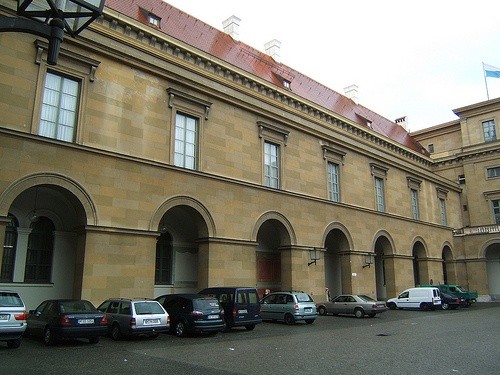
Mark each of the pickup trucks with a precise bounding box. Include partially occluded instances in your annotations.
[386,287,441,312]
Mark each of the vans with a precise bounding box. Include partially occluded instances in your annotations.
[198,286,263,332]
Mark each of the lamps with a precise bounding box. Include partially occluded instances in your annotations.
[362,252,374,268]
[308,247,321,266]
[30,188,40,222]
[160,217,168,234]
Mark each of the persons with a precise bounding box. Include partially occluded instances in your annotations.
[263,289,270,298]
[325,288,330,302]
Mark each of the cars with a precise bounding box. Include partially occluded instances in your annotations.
[97,298,172,341]
[440,292,464,311]
[260,290,318,326]
[316,294,387,319]
[154,294,226,336]
[429,285,478,308]
[27,299,108,346]
[0,290,27,347]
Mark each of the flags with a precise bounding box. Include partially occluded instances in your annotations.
[484,63,500,78]
